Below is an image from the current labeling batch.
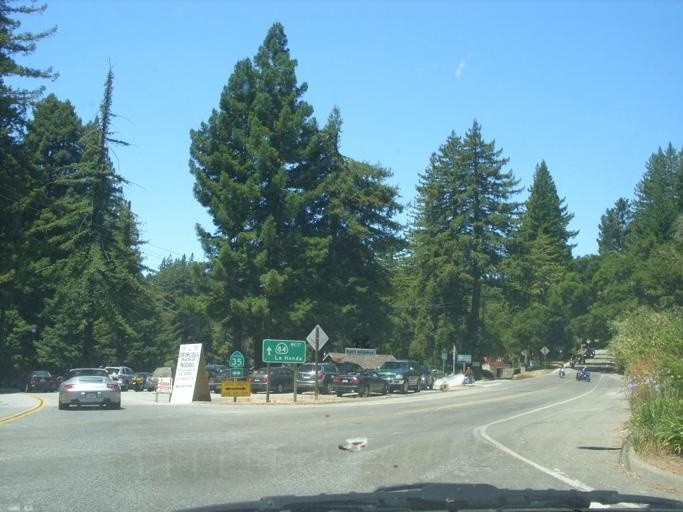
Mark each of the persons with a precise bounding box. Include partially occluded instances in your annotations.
[464,367,473,384]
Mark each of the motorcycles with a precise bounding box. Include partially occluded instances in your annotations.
[559,358,591,382]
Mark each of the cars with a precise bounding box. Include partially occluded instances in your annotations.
[206,359,433,397]
[104,367,175,392]
[21,370,55,393]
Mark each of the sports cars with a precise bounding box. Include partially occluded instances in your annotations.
[59,368,121,410]
